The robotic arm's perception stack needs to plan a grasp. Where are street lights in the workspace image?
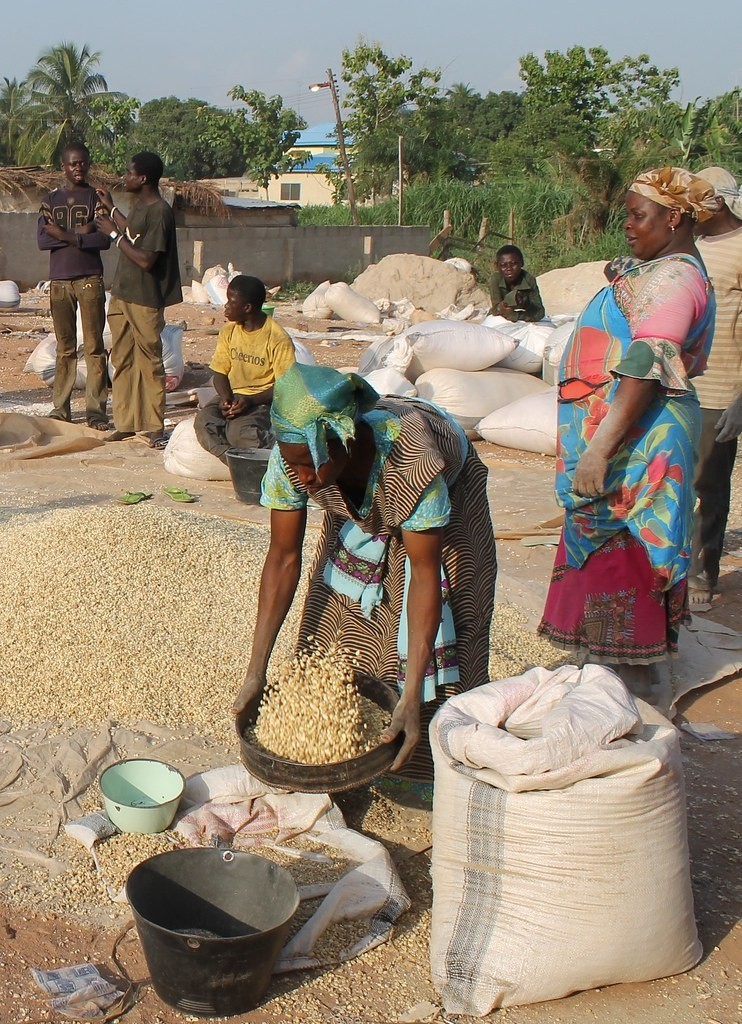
[308,69,360,227]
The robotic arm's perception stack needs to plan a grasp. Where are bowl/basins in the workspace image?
[236,675,405,793]
[98,759,186,835]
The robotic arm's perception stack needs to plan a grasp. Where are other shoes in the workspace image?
[89,420,111,431]
[102,431,136,442]
[150,434,169,450]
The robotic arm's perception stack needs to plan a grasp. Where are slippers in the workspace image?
[159,485,195,502]
[115,491,153,505]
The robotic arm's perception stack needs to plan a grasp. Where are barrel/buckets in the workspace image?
[225,448,273,504]
[262,305,275,318]
[125,849,300,1017]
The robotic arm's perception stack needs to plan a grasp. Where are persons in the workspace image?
[194,275,296,466]
[689,165,742,604]
[234,362,498,782]
[96,152,183,449]
[536,167,716,697]
[486,245,545,323]
[37,142,111,431]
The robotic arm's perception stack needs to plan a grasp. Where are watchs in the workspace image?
[108,229,118,242]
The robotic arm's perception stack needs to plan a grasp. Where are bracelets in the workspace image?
[111,207,117,218]
[115,235,123,247]
